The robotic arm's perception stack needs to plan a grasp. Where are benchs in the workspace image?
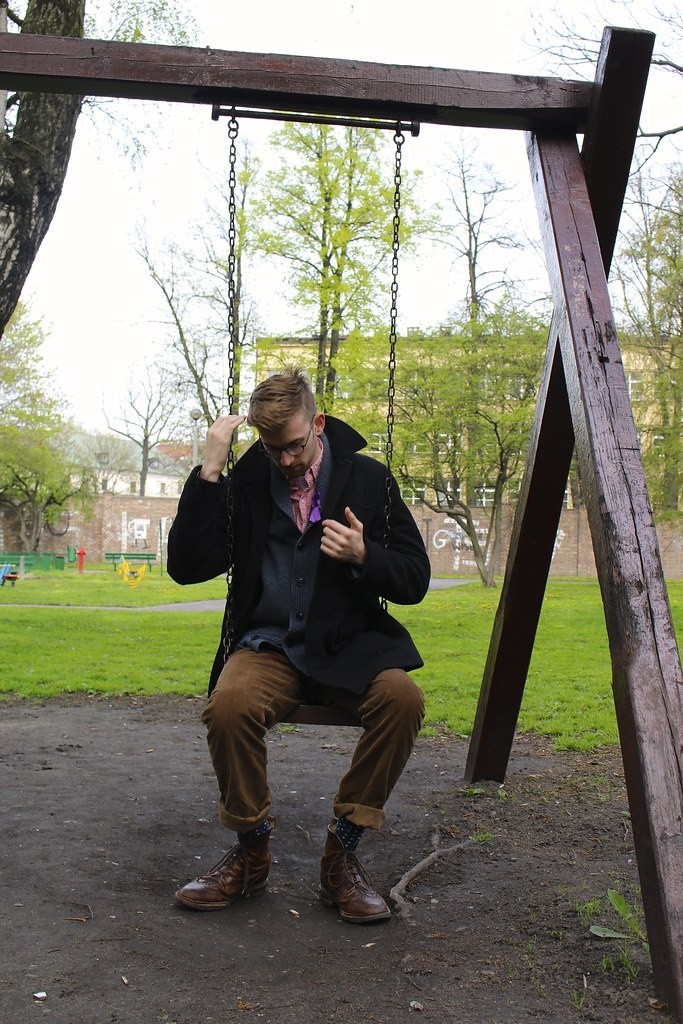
[104,552,157,573]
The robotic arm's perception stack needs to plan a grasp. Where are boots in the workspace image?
[319,815,391,922]
[172,814,276,910]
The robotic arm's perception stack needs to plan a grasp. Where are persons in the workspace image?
[164,366,432,924]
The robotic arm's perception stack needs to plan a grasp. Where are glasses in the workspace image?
[258,413,316,457]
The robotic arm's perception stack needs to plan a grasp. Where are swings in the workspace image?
[222,117,406,727]
